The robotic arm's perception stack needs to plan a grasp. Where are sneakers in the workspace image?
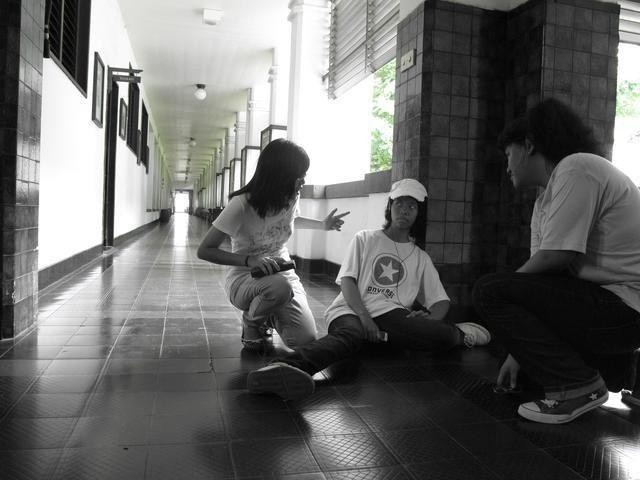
[455,322,492,349]
[517,369,609,424]
[247,362,316,400]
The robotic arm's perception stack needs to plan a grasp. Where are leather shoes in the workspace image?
[260,324,272,336]
[241,316,265,352]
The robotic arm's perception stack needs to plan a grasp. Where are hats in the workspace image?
[390,179,428,202]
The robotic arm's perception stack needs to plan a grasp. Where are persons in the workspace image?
[197,137,351,350]
[470,97,640,426]
[247,177,492,400]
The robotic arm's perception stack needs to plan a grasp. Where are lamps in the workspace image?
[194,84,207,100]
[189,137,196,147]
[187,159,192,165]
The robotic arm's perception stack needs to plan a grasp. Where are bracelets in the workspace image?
[245,255,250,266]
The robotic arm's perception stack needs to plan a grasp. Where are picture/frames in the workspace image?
[146,146,149,174]
[92,51,105,128]
[137,129,142,165]
[119,98,128,140]
